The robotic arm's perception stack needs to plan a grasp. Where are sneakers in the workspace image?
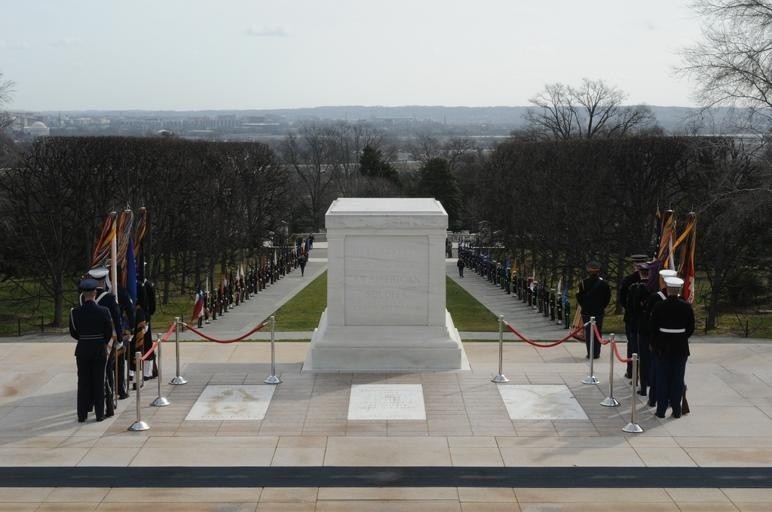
[77,368,158,423]
[624,372,681,418]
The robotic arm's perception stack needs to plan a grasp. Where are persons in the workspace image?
[67,260,160,421]
[194,248,309,328]
[457,244,571,329]
[309,232,314,250]
[619,253,697,419]
[575,259,612,358]
[447,241,452,258]
[446,237,449,246]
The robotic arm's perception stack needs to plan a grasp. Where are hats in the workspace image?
[79,278,99,292]
[586,261,602,273]
[662,276,684,289]
[658,269,678,278]
[88,268,110,280]
[637,263,651,271]
[630,254,649,262]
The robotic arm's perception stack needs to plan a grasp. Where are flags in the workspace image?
[89,206,148,301]
[654,207,696,304]
